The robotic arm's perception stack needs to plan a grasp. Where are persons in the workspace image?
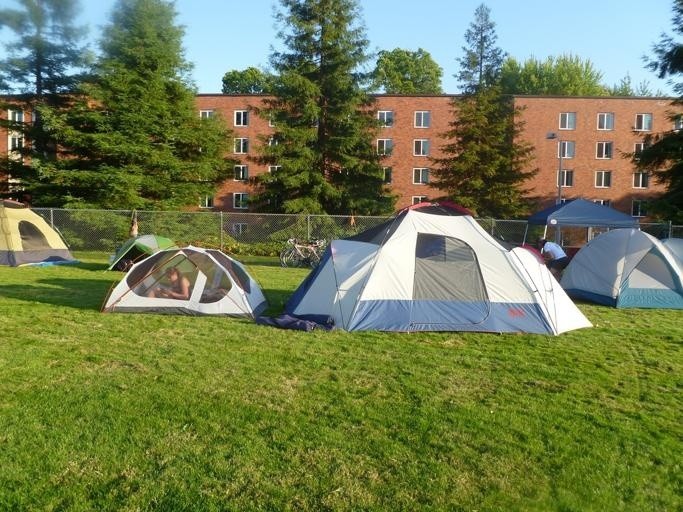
[154,265,226,299]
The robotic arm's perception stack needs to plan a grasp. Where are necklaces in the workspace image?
[537,237,570,275]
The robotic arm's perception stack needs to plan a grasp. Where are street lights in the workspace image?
[544,131,562,244]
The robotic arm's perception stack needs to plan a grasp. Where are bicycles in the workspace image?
[279,237,326,269]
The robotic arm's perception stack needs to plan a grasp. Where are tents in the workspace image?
[282,202,593,337]
[559,227,683,308]
[0,198,74,266]
[100,244,270,320]
[108,233,178,271]
